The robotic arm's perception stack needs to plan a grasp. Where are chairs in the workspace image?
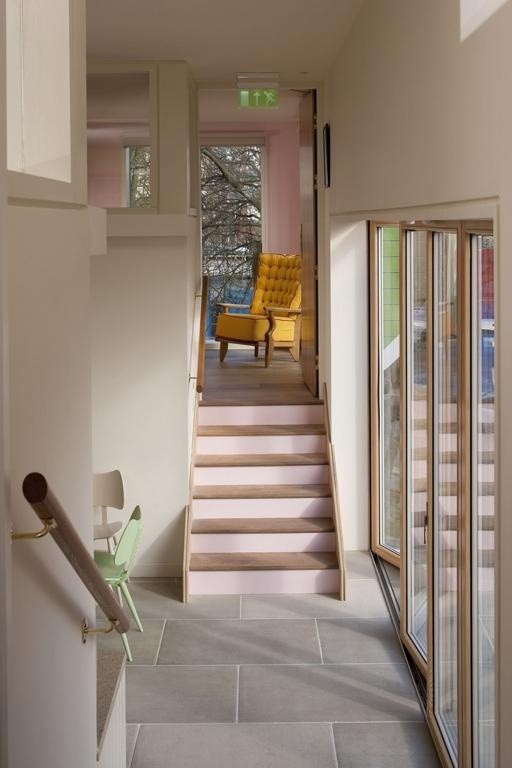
[214,253,302,367]
[94,470,144,663]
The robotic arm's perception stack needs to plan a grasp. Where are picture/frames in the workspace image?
[323,124,330,187]
